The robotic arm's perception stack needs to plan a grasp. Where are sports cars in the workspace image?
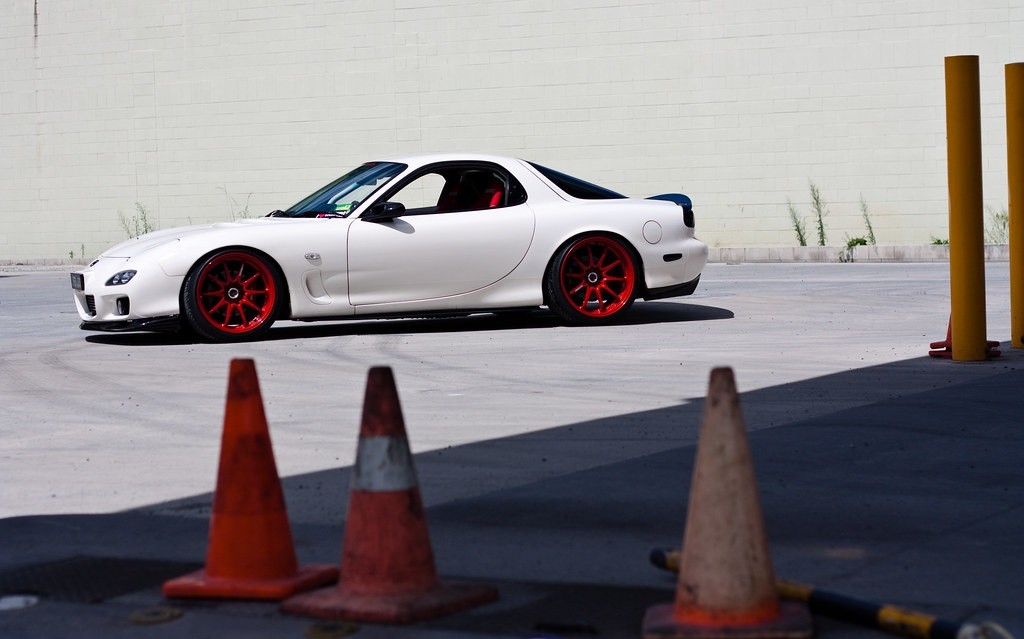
[70,154,709,342]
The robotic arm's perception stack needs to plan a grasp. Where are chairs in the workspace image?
[477,183,501,207]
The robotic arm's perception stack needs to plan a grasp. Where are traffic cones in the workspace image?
[278,365,498,626]
[641,365,814,639]
[161,358,339,601]
